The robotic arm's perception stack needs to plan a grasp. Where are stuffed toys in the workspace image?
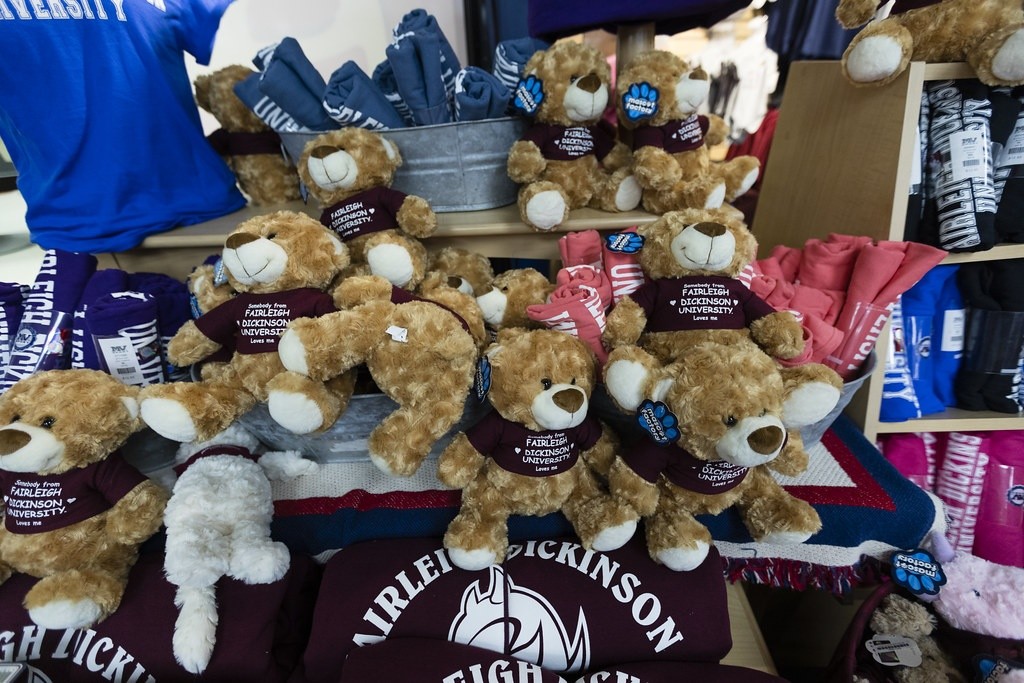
[2,41,843,675]
[835,0,1024,82]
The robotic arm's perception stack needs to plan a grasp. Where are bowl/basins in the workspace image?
[234,391,476,463]
[802,346,880,455]
[272,115,533,213]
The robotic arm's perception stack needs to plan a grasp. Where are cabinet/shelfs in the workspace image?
[90,56,1024,683]
